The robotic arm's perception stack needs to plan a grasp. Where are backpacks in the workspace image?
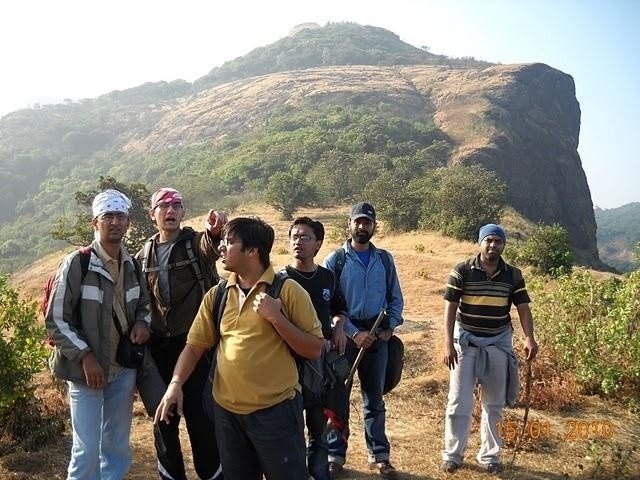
[212,274,325,409]
[41,247,91,347]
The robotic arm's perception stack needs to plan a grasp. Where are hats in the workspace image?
[478,223,506,245]
[152,187,183,209]
[92,189,132,219]
[350,202,376,223]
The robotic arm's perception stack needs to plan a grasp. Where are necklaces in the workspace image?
[289,264,319,280]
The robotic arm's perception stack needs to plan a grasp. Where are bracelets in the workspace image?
[351,330,360,338]
[170,380,182,386]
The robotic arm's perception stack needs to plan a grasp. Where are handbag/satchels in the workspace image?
[325,347,353,393]
[110,304,144,369]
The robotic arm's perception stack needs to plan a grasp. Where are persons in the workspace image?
[153,217,324,480]
[441,224,538,475]
[136,188,228,480]
[322,203,404,479]
[45,191,151,480]
[276,216,349,480]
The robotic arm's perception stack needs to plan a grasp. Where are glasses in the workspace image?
[95,212,127,222]
[153,202,182,209]
[219,238,243,246]
[291,234,317,241]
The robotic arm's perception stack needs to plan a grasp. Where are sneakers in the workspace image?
[369,461,396,477]
[441,461,457,472]
[485,462,501,472]
[329,462,343,478]
